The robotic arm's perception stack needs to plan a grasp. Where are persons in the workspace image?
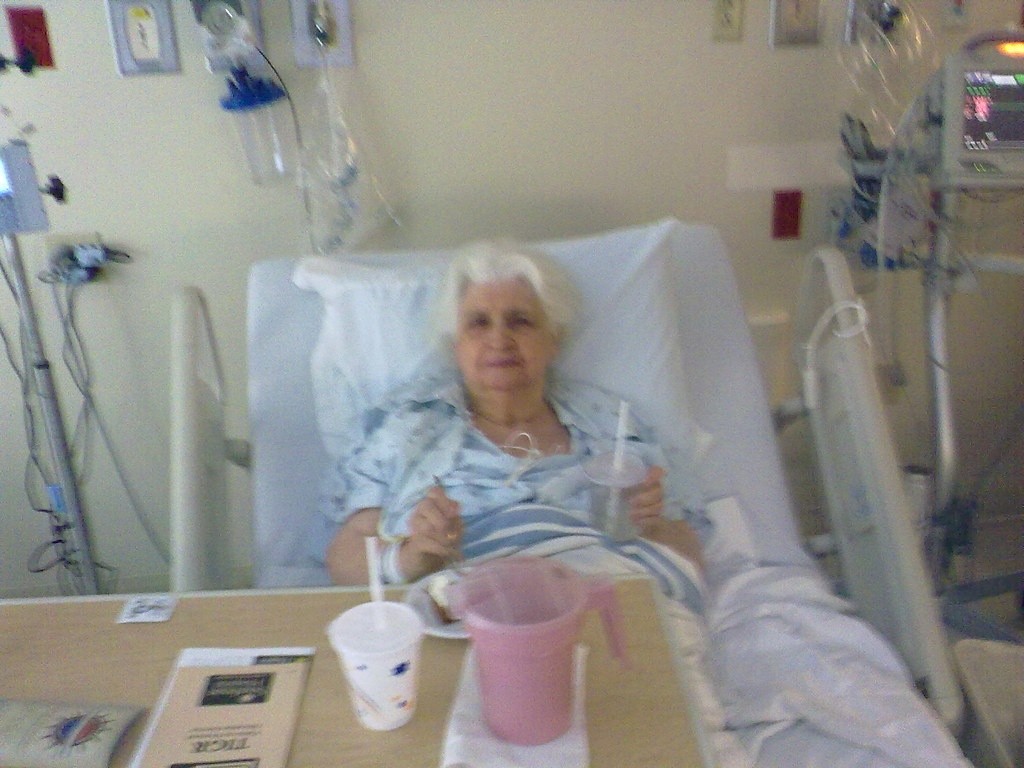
[318,236,706,584]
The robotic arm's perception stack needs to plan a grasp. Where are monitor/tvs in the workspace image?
[943,51,1024,193]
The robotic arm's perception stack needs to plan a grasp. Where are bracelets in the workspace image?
[380,532,409,586]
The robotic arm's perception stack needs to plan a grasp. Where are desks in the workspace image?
[0,574,703,767]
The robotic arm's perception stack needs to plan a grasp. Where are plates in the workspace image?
[407,566,480,640]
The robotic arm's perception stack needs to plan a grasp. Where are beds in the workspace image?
[166,216,977,768]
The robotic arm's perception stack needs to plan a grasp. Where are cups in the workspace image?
[584,450,648,541]
[456,557,634,746]
[326,599,423,733]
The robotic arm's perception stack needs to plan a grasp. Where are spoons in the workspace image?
[428,472,467,575]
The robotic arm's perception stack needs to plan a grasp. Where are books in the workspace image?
[129,646,317,768]
